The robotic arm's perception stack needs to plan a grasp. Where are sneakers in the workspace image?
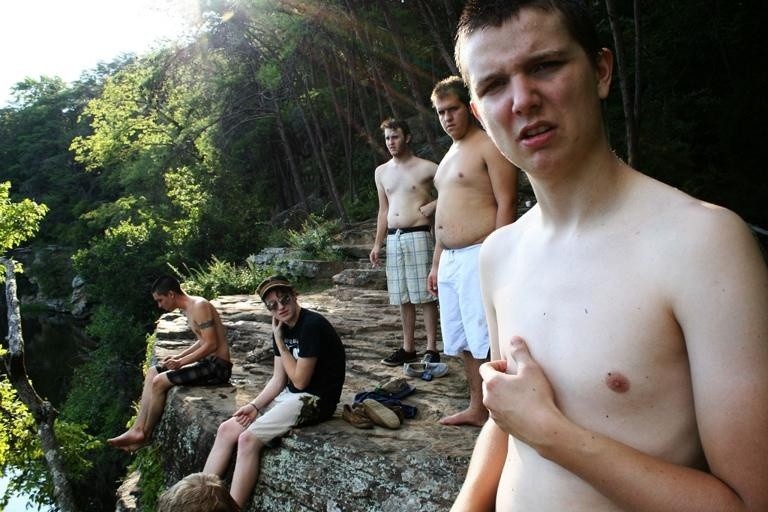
[342,404,373,429]
[406,363,448,378]
[363,399,400,430]
[381,348,417,367]
[423,351,440,364]
[387,406,403,425]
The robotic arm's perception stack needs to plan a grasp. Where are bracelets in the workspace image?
[248,403,259,412]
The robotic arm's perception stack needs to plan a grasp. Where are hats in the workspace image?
[376,377,416,401]
[256,275,292,300]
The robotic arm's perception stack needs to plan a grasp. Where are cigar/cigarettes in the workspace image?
[371,261,378,270]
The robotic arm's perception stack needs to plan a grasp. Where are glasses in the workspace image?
[265,296,291,312]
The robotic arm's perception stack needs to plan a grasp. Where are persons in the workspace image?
[107,275,233,451]
[369,119,440,366]
[154,472,242,512]
[447,1,768,512]
[427,76,518,427]
[203,276,345,510]
